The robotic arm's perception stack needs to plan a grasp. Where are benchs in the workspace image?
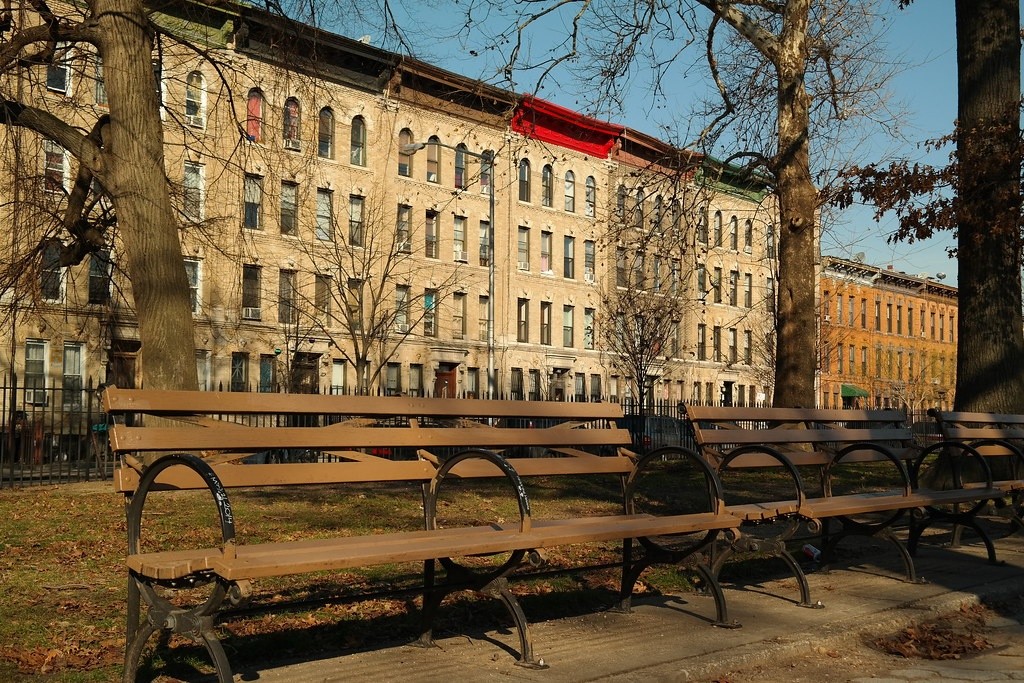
[927,408,1024,567]
[103,385,742,683]
[677,403,1008,608]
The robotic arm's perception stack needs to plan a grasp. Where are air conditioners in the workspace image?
[396,243,413,254]
[395,324,409,333]
[243,307,262,320]
[282,139,301,150]
[454,252,467,262]
[518,261,530,272]
[26,390,49,405]
[186,114,205,129]
[585,273,597,283]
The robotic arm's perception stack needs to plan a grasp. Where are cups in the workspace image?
[802,544,821,562]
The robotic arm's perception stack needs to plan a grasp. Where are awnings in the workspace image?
[841,384,869,398]
